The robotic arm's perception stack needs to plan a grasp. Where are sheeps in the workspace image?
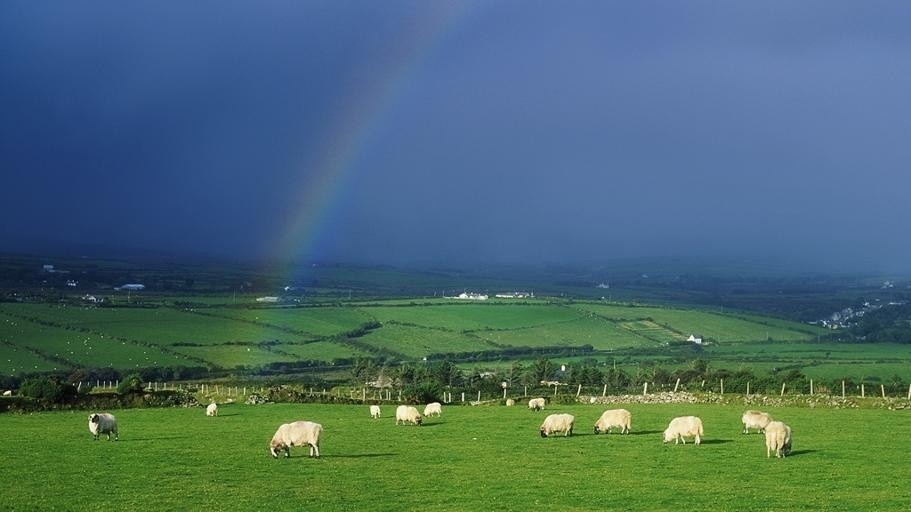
[206,402,219,417]
[269,420,324,459]
[741,410,774,434]
[421,402,442,418]
[87,413,118,441]
[765,420,792,458]
[395,405,423,427]
[370,405,381,419]
[528,397,546,411]
[662,415,704,446]
[506,398,515,407]
[593,408,632,435]
[539,413,575,438]
